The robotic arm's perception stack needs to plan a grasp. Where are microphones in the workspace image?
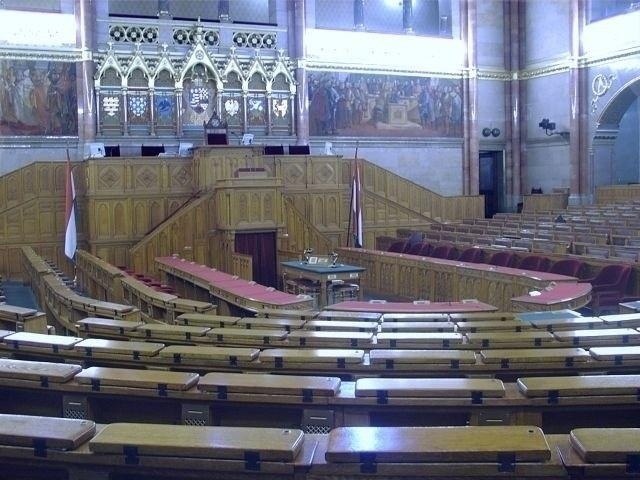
[231,132,240,145]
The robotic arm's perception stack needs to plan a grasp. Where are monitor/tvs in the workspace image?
[83,143,106,159]
[241,134,254,145]
[324,141,333,155]
[178,143,194,157]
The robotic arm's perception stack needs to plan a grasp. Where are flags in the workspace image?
[64,159,77,259]
[352,161,363,247]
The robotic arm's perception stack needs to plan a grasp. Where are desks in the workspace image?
[0,246,640,480]
[375,199,640,246]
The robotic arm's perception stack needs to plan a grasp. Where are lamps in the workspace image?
[538,117,570,138]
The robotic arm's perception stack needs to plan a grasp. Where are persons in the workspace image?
[2,59,77,135]
[306,71,463,139]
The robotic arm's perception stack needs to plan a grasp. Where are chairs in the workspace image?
[140,142,165,157]
[264,144,310,156]
[103,145,121,157]
[206,132,229,147]
